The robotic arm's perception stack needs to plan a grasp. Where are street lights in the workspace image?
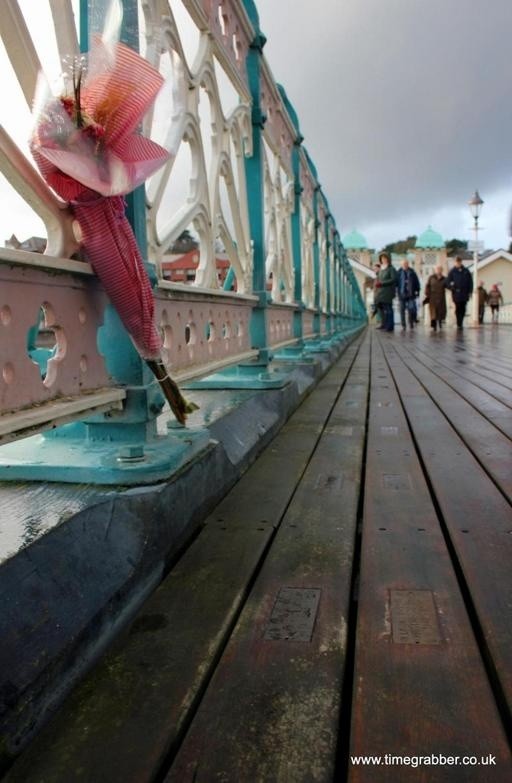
[466,190,486,328]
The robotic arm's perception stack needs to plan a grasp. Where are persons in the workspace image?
[371,250,397,332]
[486,284,504,323]
[423,265,461,333]
[477,280,488,324]
[446,253,473,330]
[394,257,421,332]
[372,263,384,329]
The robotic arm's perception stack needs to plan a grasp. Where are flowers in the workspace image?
[31,55,200,425]
